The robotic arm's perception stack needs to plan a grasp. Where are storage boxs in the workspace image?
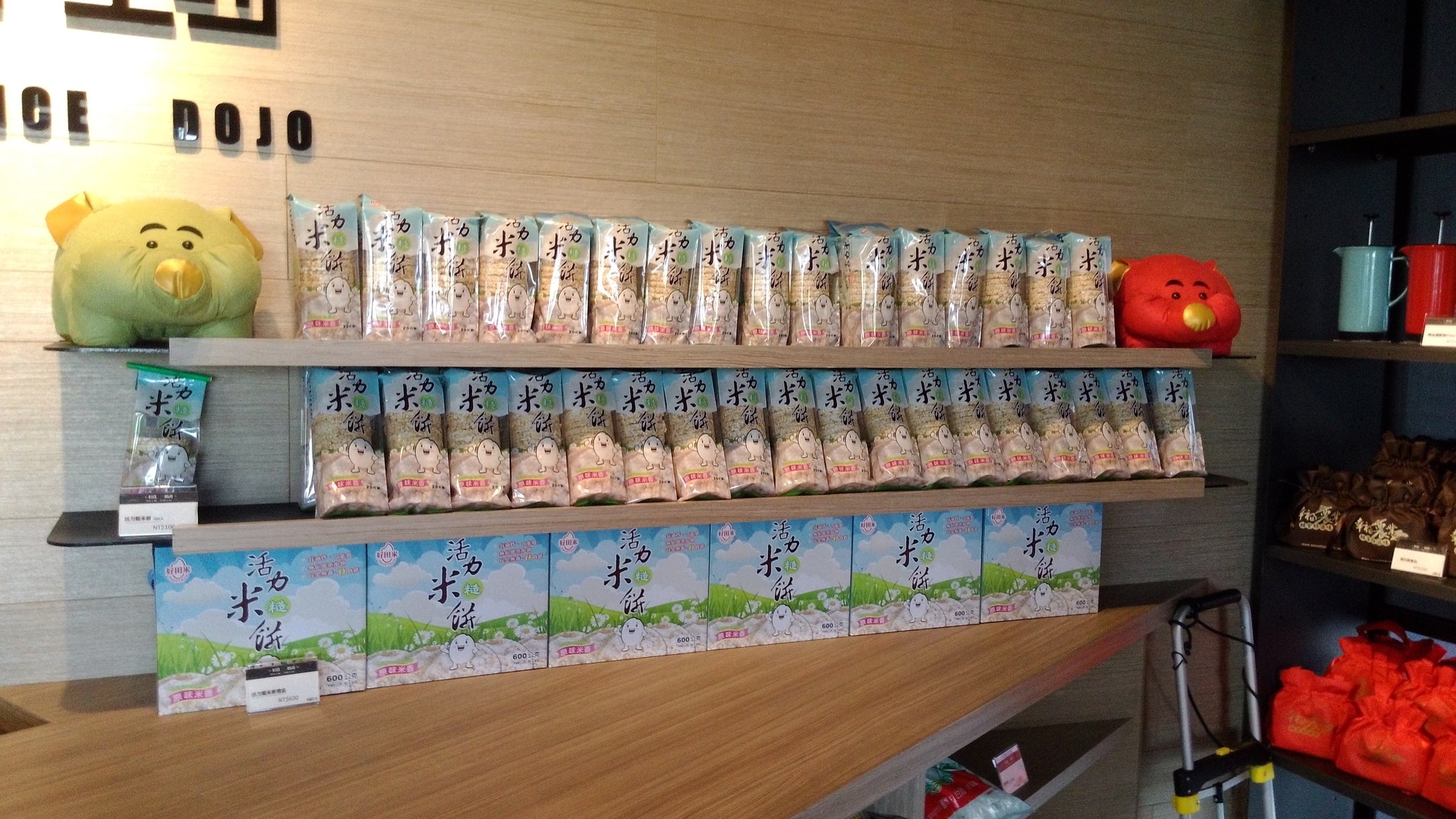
[151,539,368,715]
[366,535,551,693]
[848,508,983,638]
[981,503,1103,624]
[708,517,854,652]
[549,524,709,670]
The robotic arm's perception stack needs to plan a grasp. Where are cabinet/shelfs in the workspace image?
[1,577,1210,819]
[45,331,1259,555]
[1258,109,1456,819]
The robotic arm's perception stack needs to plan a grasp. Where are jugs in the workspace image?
[1400,245,1455,335]
[1333,245,1408,334]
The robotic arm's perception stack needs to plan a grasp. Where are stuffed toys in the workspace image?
[49,189,265,346]
[1110,254,1241,356]
